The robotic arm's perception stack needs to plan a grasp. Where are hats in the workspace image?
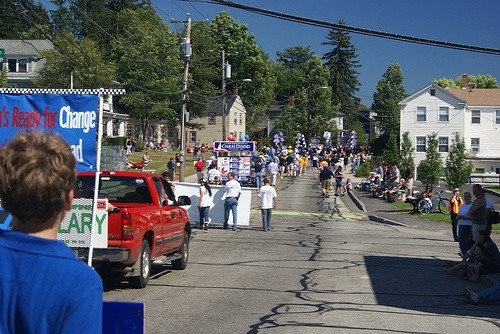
[453,188,460,192]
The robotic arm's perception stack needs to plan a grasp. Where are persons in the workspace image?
[187,140,414,201]
[223,172,241,231]
[259,178,278,232]
[141,152,152,164]
[464,281,500,305]
[162,171,175,194]
[0,128,104,334]
[417,188,433,212]
[444,184,500,281]
[175,153,180,172]
[197,178,212,230]
[148,139,180,151]
[126,136,137,154]
[125,157,136,164]
[167,158,176,181]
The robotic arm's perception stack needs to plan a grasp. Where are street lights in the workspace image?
[221,78,253,141]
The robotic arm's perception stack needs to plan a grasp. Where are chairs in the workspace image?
[124,192,142,199]
[136,186,150,199]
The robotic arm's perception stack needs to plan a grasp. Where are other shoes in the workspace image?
[469,274,479,282]
[223,226,228,229]
[463,275,471,279]
[199,226,203,229]
[232,228,237,231]
[264,228,272,232]
[446,268,456,273]
[204,225,208,230]
[341,194,345,196]
[334,193,339,196]
[458,273,466,278]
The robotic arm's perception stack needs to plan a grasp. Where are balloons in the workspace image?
[262,162,266,166]
[245,135,249,139]
[240,137,243,141]
[227,136,236,141]
[267,157,271,161]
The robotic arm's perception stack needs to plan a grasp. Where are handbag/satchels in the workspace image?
[489,211,500,224]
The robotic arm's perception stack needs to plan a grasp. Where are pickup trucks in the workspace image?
[54,170,193,290]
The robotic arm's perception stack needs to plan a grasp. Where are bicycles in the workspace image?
[417,190,451,216]
